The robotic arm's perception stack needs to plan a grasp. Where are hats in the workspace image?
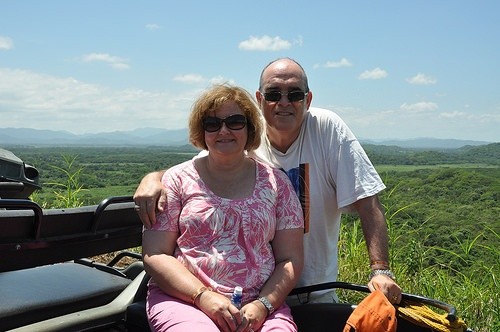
[342,289,397,332]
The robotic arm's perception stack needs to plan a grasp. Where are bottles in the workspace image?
[227,285,244,329]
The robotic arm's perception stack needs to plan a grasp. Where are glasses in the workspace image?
[260,90,308,102]
[202,114,247,133]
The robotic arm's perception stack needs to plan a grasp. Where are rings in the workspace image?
[135,204,140,212]
[250,329,254,332]
[392,295,398,300]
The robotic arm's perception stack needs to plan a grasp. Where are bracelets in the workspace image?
[191,287,207,305]
[370,260,389,266]
[256,296,275,316]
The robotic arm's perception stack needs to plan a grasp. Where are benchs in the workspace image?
[0,190,139,330]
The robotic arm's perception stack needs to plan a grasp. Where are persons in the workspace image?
[141,85,305,332]
[132,57,402,306]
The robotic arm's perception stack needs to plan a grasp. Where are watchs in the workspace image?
[369,270,394,279]
